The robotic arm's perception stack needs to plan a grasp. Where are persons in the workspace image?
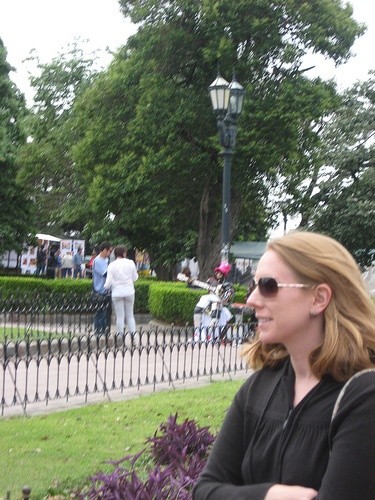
[206,265,235,307]
[91,241,138,336]
[33,242,100,279]
[192,231,375,500]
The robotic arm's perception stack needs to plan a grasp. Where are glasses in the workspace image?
[252,277,313,297]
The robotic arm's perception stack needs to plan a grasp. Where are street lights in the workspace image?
[208,62,246,266]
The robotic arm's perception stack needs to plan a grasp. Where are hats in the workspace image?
[212,261,232,278]
